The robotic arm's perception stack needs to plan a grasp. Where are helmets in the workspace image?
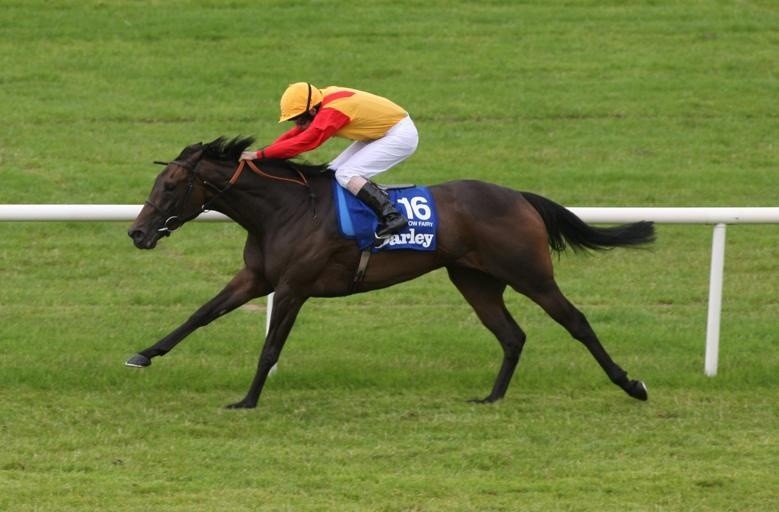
[278,82,323,123]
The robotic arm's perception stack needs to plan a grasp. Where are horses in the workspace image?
[124,134,657,409]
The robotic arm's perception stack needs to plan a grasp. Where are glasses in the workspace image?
[294,83,314,126]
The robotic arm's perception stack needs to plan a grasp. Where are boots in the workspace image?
[356,181,408,237]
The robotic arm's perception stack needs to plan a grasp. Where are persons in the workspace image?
[237,81,419,236]
